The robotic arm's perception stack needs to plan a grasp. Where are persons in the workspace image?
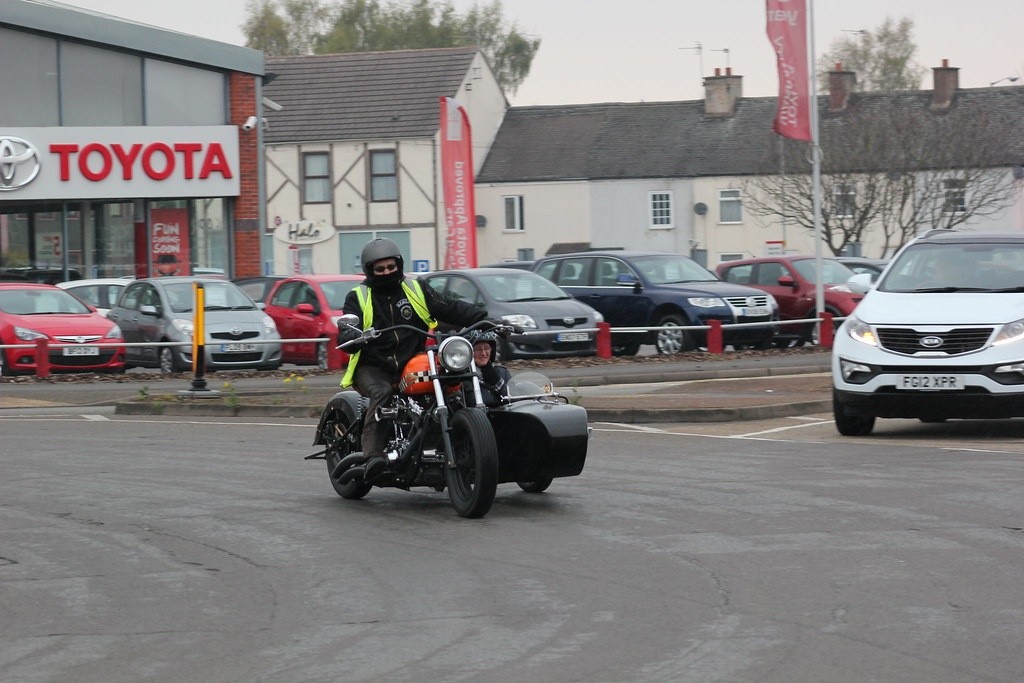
[457,329,513,407]
[335,238,526,480]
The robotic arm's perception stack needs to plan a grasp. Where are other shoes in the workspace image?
[366,457,389,486]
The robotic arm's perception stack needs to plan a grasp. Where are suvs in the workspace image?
[830,228,1024,437]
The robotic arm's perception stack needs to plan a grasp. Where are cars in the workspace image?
[228,277,334,306]
[37,278,156,318]
[822,258,890,282]
[108,275,284,374]
[532,251,779,354]
[716,256,868,347]
[420,268,604,361]
[0,281,127,374]
[265,274,368,372]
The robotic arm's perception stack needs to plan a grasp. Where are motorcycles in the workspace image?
[303,314,592,521]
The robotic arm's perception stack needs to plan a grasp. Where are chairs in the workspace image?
[178,292,191,310]
[560,263,664,283]
[457,281,509,303]
[732,265,785,282]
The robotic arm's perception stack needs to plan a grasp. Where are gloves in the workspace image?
[339,329,368,351]
[481,364,505,392]
[445,371,466,387]
[484,317,524,339]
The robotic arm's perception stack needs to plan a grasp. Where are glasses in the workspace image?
[373,263,398,272]
[474,346,492,354]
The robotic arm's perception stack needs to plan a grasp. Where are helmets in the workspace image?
[361,237,404,281]
[463,330,498,364]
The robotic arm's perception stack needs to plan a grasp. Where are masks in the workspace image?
[371,272,401,289]
